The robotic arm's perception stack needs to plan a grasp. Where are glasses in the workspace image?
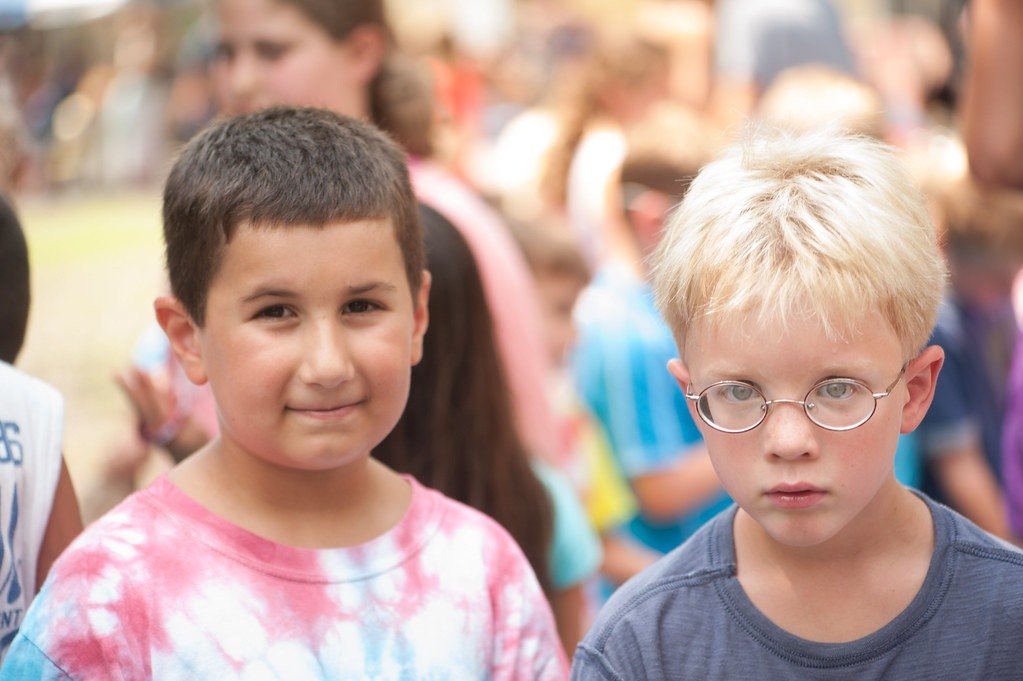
[685,364,908,435]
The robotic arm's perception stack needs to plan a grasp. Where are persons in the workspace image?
[0,0,1023,681]
